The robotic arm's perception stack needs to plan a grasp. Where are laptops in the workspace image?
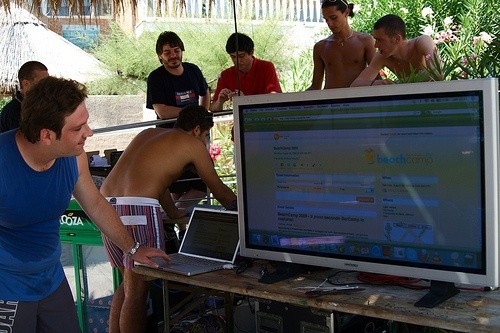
[134,207,241,277]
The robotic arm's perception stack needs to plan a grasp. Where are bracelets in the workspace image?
[127,241,140,256]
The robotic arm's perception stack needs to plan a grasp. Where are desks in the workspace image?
[161,204,228,224]
[58,200,123,333]
[133,263,500,333]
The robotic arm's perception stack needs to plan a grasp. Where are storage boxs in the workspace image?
[254,299,391,333]
[70,295,114,333]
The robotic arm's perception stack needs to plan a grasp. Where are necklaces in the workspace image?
[340,30,354,46]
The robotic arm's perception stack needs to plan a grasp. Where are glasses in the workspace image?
[204,112,213,119]
[320,0,348,9]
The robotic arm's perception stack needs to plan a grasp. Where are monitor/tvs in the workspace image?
[231,77,500,309]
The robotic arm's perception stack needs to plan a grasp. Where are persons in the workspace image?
[350,14,443,87]
[306,0,382,91]
[209,32,282,112]
[0,31,240,333]
